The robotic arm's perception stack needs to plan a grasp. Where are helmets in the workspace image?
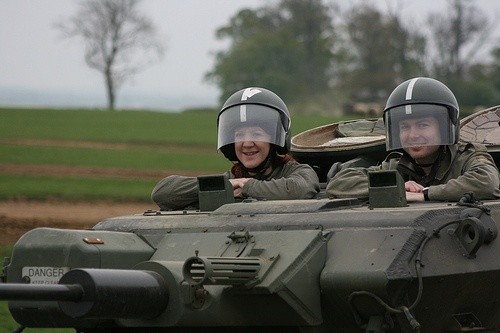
[383,77,460,151]
[217,86,291,161]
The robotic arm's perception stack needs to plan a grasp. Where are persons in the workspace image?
[151,87,319,211]
[325,77,500,202]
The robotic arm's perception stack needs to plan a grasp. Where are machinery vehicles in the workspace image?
[0,103,500,333]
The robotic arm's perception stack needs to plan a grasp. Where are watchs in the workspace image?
[422,187,430,201]
